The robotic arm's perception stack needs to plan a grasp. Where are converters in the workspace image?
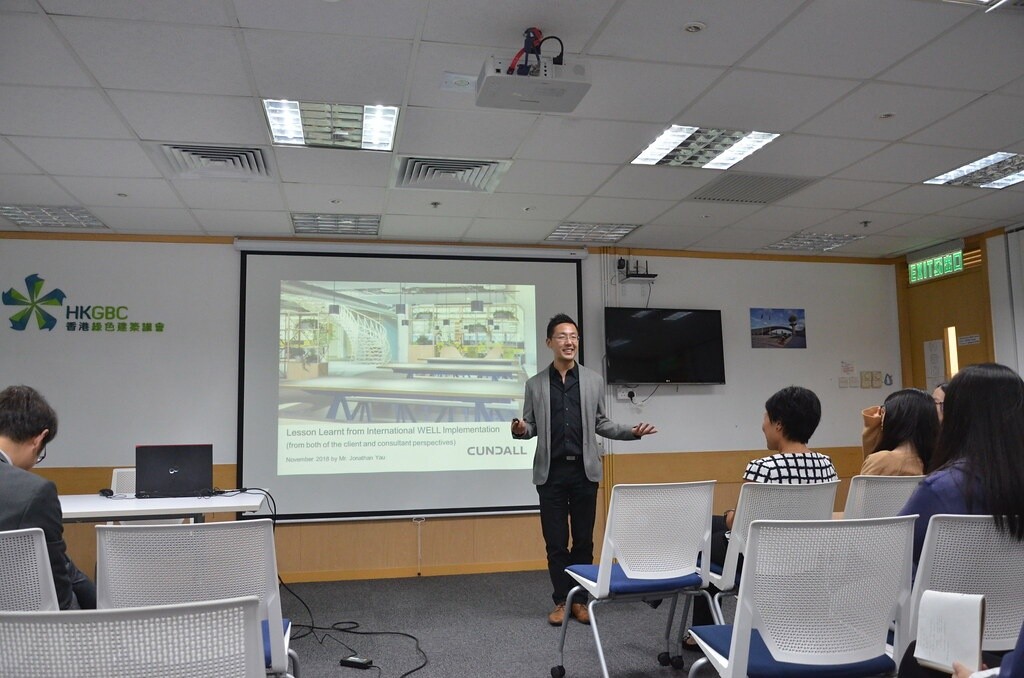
[339,656,372,668]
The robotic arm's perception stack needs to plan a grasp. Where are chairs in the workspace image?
[0,519,301,678]
[105,468,195,524]
[551,475,1023,678]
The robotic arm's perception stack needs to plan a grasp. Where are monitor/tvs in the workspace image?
[604,307,725,385]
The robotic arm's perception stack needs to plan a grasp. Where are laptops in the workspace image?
[135,445,225,499]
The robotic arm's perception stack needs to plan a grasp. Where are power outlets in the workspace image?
[616,388,634,400]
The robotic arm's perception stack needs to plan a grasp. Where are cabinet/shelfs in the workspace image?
[281,310,325,377]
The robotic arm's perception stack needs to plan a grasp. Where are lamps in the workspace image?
[329,280,501,331]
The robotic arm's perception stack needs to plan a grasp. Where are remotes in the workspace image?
[513,418,519,423]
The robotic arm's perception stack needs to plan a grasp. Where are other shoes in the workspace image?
[682,634,701,651]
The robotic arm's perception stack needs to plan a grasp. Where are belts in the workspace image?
[563,455,580,461]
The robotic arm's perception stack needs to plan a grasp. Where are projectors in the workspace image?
[473,54,591,113]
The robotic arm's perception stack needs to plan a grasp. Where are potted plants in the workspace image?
[416,312,516,346]
[287,354,328,383]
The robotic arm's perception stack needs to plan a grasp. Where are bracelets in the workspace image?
[724,509,735,522]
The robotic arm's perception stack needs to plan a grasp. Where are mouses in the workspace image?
[99,489,114,496]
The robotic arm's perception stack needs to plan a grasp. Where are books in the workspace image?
[913,590,987,676]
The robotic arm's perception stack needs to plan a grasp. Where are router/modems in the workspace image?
[625,259,657,278]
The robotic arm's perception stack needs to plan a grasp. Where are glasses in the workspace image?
[36,441,47,463]
[939,402,943,412]
[550,335,579,342]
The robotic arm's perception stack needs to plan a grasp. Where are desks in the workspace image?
[57,488,270,523]
[284,358,526,423]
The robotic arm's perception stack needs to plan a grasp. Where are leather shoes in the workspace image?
[572,603,596,624]
[548,601,566,625]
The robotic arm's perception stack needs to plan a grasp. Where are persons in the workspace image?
[682,387,837,652]
[897,620,1024,678]
[860,383,949,476]
[895,363,1024,570]
[510,314,658,625]
[0,385,97,611]
